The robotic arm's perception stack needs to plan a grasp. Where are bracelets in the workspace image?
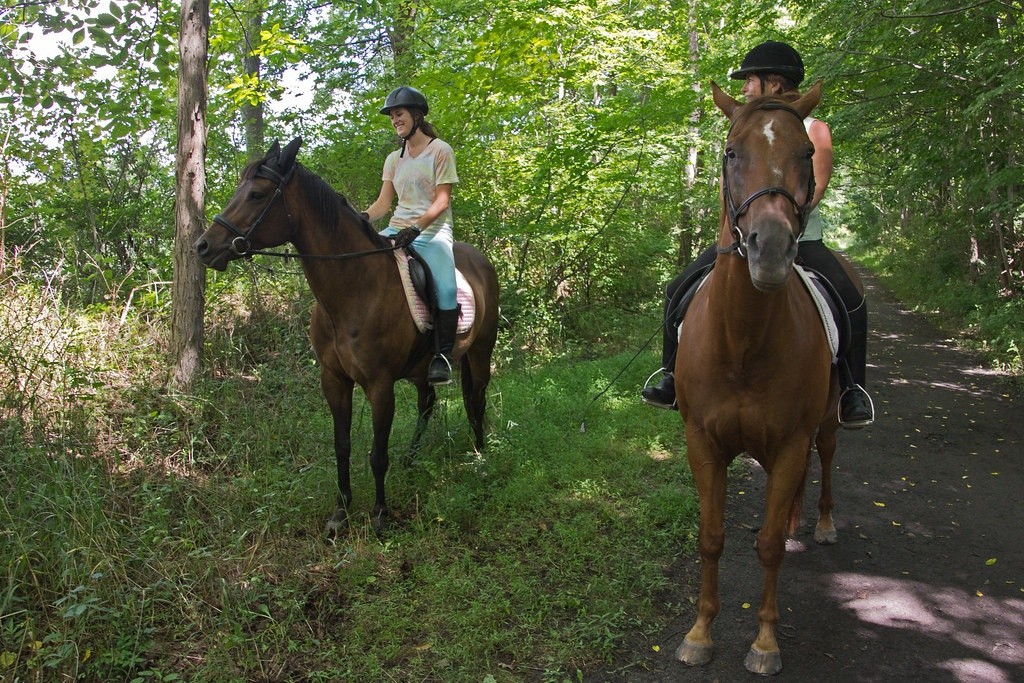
[413,224,423,232]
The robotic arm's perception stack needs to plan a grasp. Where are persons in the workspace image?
[641,39,874,430]
[357,86,461,383]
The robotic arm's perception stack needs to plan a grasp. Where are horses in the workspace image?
[670,75,868,678]
[193,135,501,548]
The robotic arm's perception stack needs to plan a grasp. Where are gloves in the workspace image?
[395,226,421,250]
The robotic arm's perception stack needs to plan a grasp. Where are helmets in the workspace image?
[379,87,429,117]
[729,40,804,82]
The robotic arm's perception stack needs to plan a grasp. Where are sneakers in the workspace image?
[641,374,678,411]
[839,390,871,430]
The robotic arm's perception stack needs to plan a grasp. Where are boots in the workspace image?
[426,303,462,383]
